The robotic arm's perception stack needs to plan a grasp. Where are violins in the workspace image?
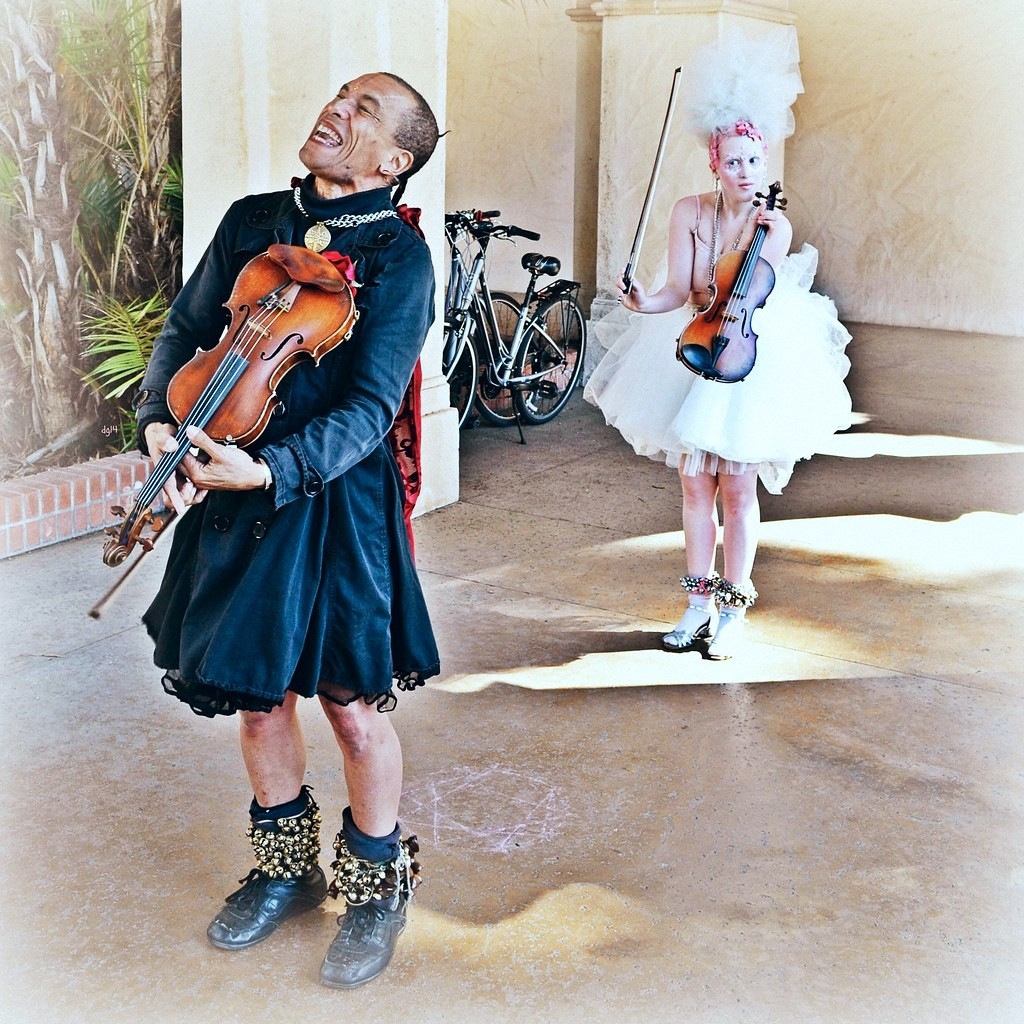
[675,180,788,383]
[103,243,359,571]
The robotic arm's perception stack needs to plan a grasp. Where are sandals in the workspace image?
[710,611,749,661]
[661,604,719,650]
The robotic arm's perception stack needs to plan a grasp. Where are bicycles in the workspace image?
[444,209,587,445]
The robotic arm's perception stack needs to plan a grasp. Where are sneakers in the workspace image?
[319,891,407,990]
[206,867,328,951]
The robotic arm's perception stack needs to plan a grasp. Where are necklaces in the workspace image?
[707,189,759,298]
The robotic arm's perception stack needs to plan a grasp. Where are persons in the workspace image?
[133,73,441,988]
[583,32,853,663]
